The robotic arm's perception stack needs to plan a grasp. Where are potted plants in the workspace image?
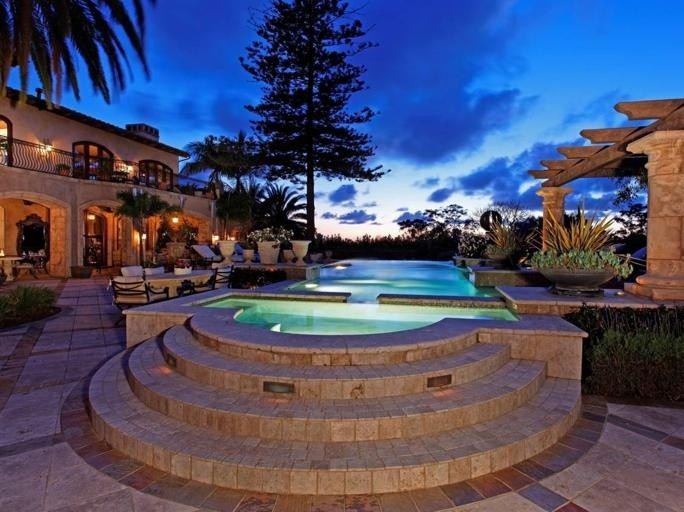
[55,163,71,176]
[483,197,646,291]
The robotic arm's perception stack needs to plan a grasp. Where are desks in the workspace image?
[0,256,24,281]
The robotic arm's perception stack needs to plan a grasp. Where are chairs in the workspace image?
[16,257,39,280]
[110,264,234,326]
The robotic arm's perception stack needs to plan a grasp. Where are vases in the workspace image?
[283,240,312,267]
[256,241,280,264]
[216,240,238,265]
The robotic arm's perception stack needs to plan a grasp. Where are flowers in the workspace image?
[174,260,191,269]
[247,225,295,245]
